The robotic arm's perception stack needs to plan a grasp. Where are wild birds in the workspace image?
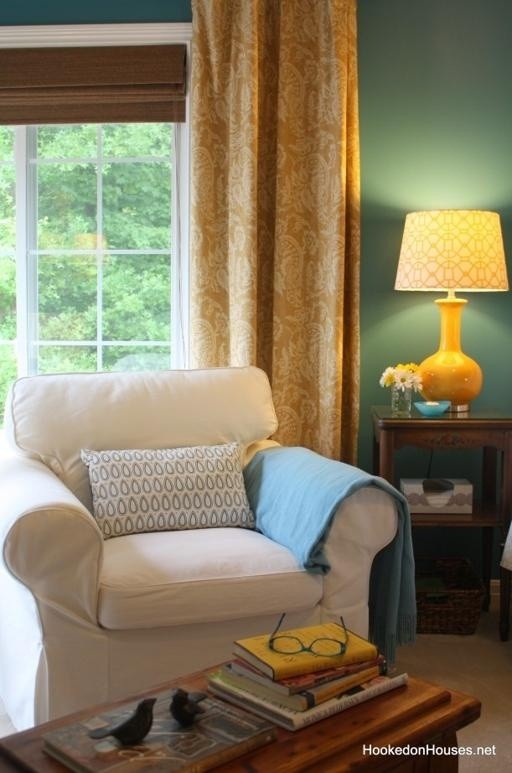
[170,686,209,729]
[89,696,157,744]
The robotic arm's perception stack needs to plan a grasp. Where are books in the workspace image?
[42,687,279,773]
[206,621,410,733]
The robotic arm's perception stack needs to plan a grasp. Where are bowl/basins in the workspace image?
[413,400,452,417]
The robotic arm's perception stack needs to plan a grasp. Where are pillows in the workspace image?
[78,431,254,541]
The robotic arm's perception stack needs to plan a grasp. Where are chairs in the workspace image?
[0,360,410,725]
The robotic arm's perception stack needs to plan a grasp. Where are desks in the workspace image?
[366,403,509,645]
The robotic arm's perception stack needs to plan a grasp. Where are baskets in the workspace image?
[414,557,488,635]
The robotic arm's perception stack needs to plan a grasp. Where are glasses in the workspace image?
[270,614,348,656]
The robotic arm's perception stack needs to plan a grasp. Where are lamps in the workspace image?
[389,205,512,415]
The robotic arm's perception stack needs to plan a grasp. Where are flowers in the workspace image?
[378,361,422,413]
